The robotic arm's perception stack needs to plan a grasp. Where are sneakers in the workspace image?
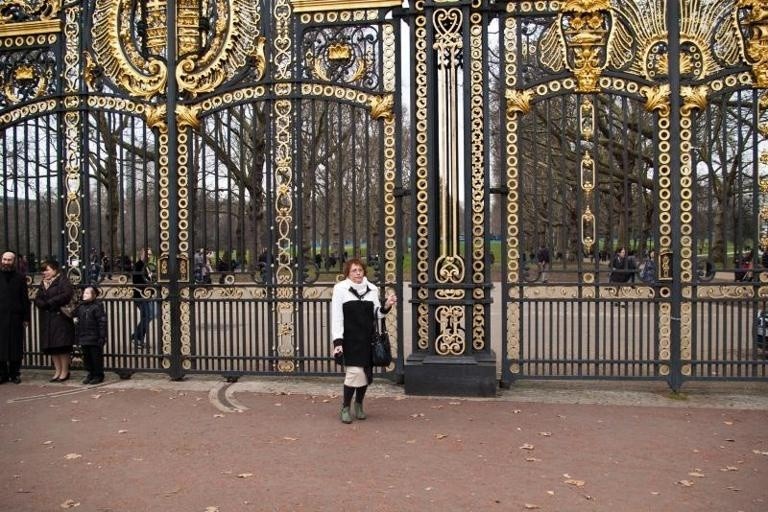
[131,339,147,348]
[82,377,103,384]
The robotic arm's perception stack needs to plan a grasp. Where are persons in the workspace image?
[331,259,398,423]
[0,245,156,385]
[523,240,658,309]
[315,252,348,273]
[734,248,760,282]
[194,247,278,284]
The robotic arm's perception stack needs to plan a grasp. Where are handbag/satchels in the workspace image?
[371,333,392,367]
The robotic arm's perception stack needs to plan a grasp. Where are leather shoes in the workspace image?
[354,399,366,419]
[0,375,21,384]
[48,372,69,382]
[340,403,352,423]
